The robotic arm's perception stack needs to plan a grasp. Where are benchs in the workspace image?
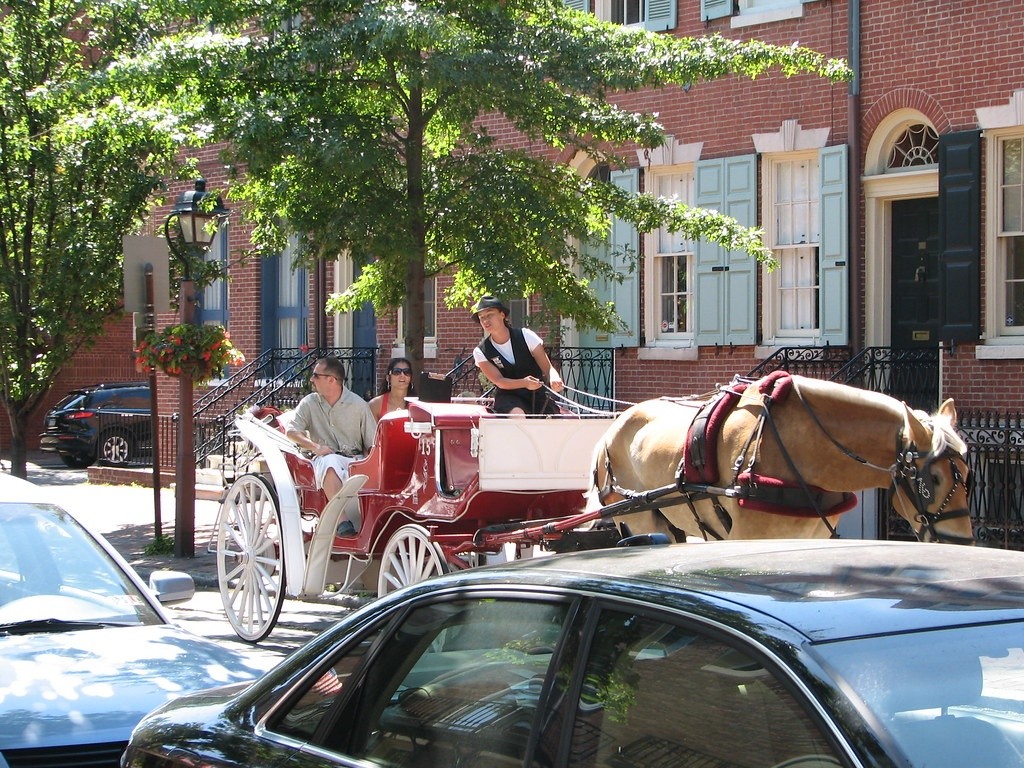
[277,405,479,536]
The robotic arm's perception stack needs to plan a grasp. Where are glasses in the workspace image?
[388,368,412,377]
[312,373,337,380]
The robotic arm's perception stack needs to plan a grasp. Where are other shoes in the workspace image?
[336,520,357,537]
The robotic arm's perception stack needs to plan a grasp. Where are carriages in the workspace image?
[206,369,976,646]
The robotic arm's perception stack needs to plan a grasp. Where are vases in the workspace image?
[164,359,212,379]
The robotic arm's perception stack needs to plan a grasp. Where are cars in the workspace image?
[0,474,275,768]
[118,533,1023,768]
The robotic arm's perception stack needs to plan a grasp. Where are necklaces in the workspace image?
[392,397,404,411]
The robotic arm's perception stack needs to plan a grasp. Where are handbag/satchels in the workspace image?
[418,372,452,403]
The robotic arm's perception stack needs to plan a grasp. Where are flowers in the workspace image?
[296,344,315,396]
[134,324,244,389]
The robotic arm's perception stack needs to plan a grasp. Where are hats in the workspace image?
[470,295,510,324]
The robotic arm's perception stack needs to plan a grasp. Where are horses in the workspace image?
[592,374,977,546]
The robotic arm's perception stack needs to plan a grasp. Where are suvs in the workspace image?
[37,381,151,468]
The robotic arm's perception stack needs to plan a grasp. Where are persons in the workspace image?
[285,357,378,537]
[470,295,564,419]
[368,358,417,425]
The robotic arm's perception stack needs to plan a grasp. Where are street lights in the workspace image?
[164,178,228,558]
